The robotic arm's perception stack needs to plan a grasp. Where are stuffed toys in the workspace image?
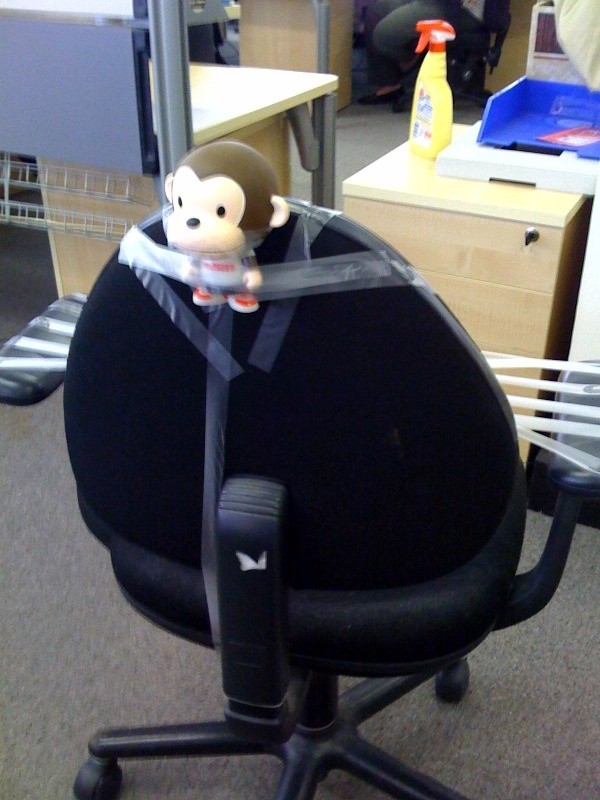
[163,140,290,314]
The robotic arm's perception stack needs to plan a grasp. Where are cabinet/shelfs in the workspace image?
[37,57,340,300]
[341,124,595,473]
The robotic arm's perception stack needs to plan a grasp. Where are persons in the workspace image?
[357,0,500,106]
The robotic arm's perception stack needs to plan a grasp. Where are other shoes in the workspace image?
[392,91,414,111]
[358,85,406,105]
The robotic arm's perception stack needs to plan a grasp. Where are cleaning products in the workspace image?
[405,18,458,165]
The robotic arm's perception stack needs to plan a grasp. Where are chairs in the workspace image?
[0,192,600,800]
